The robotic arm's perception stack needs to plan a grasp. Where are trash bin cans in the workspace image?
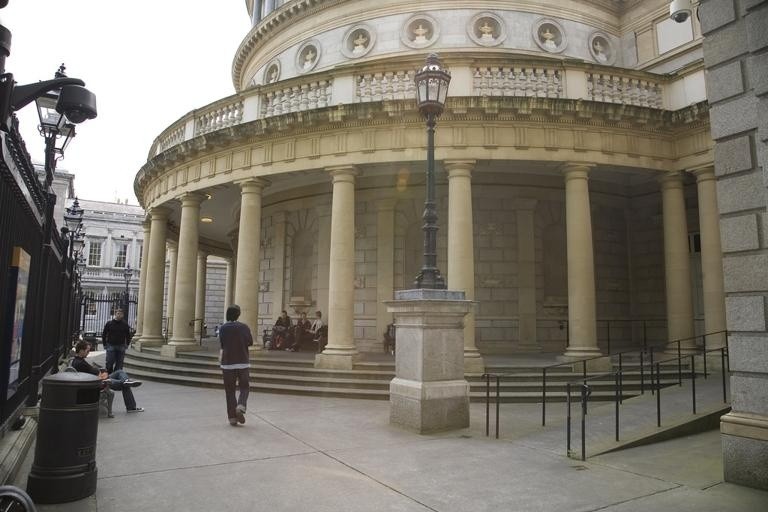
[84,331,96,351]
[26,366,102,504]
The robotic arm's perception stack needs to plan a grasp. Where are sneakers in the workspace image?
[126,405,145,413]
[123,379,142,387]
[229,409,246,426]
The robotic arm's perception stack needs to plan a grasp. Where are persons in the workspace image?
[267,310,291,350]
[71,340,145,413]
[102,305,131,375]
[291,311,323,351]
[286,313,311,351]
[384,318,395,356]
[217,304,253,426]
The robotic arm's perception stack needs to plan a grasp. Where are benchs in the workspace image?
[63,357,115,418]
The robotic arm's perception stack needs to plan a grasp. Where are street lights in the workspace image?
[34,64,77,194]
[63,198,86,275]
[413,53,453,290]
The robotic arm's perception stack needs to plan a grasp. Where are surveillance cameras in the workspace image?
[54,84,98,126]
